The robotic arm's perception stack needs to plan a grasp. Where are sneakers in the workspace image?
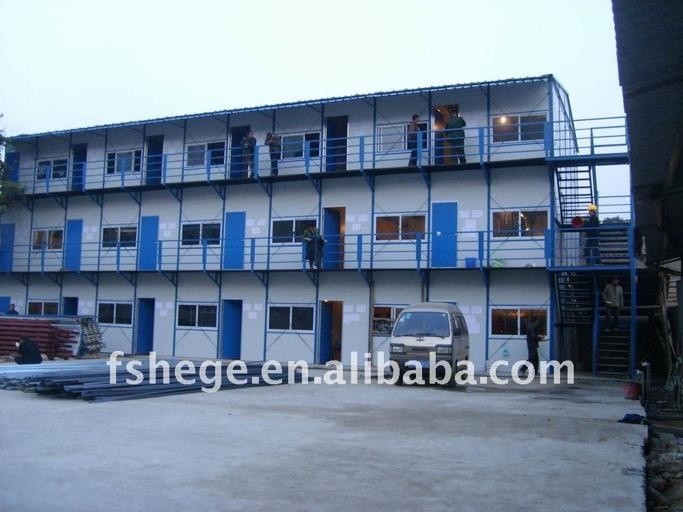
[603,328,619,332]
[518,370,540,377]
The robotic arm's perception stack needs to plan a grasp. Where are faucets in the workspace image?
[640,361,648,366]
[635,369,642,374]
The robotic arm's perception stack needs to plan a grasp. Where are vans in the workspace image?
[389,302,470,388]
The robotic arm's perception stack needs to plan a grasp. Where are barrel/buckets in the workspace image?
[624,381,641,399]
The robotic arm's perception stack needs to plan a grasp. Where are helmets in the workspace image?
[588,204,597,210]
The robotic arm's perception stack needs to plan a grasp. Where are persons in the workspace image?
[518,315,547,378]
[301,220,324,271]
[603,275,625,335]
[264,131,282,176]
[6,303,19,315]
[13,335,44,365]
[408,114,421,166]
[240,130,260,179]
[582,203,603,266]
[444,111,467,165]
